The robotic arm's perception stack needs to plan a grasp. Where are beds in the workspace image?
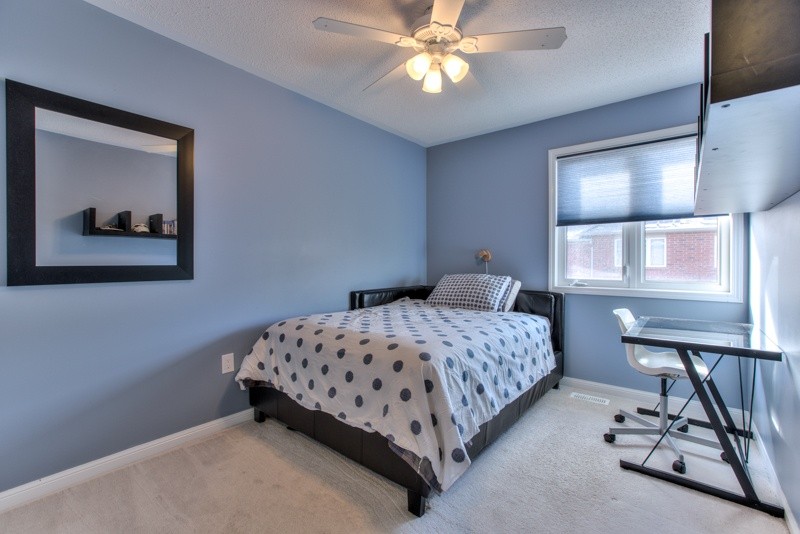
[233,285,564,517]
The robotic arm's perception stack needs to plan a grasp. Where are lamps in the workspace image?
[405,46,469,93]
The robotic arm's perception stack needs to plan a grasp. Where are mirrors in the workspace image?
[4,77,196,286]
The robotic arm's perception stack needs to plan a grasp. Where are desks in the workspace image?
[621,315,786,520]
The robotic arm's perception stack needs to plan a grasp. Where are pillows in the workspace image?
[426,273,522,313]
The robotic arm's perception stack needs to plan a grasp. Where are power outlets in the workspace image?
[221,353,234,374]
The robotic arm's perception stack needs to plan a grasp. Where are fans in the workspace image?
[312,0,568,100]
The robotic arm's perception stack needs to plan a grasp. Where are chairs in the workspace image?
[612,306,736,474]
